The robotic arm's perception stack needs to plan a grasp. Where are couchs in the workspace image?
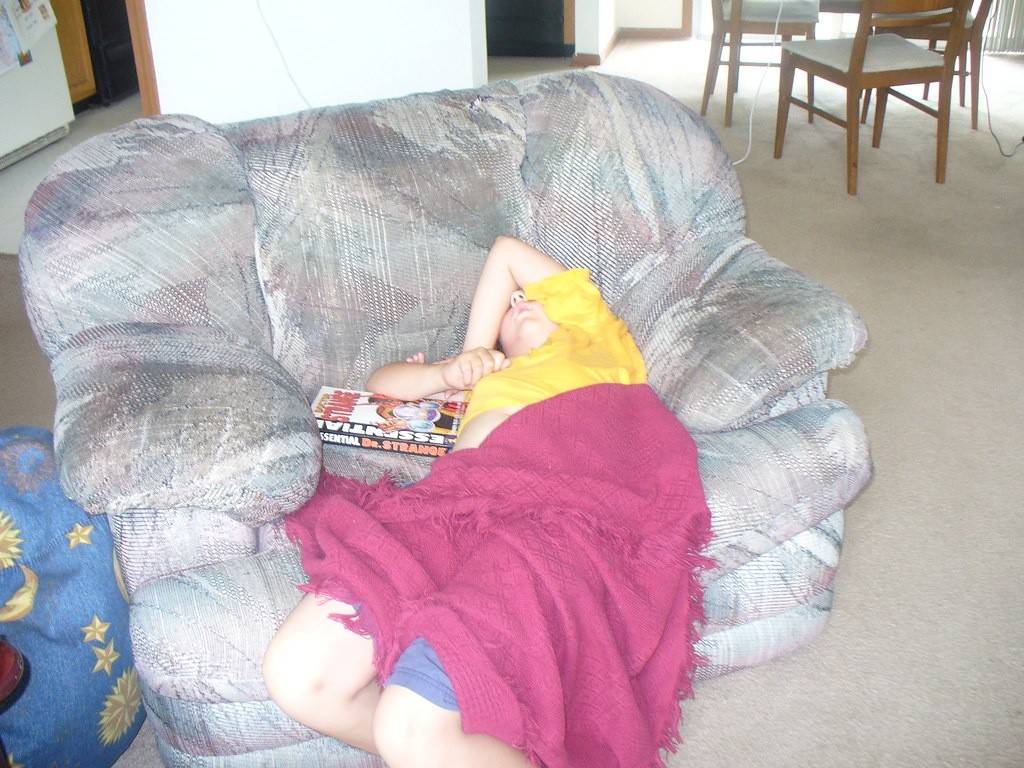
[16,69,872,768]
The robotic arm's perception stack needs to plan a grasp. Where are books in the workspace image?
[311,386,468,457]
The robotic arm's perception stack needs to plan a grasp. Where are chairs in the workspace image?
[861,1,995,131]
[698,0,818,126]
[773,1,973,195]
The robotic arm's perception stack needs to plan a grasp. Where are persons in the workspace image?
[262,235,719,767]
